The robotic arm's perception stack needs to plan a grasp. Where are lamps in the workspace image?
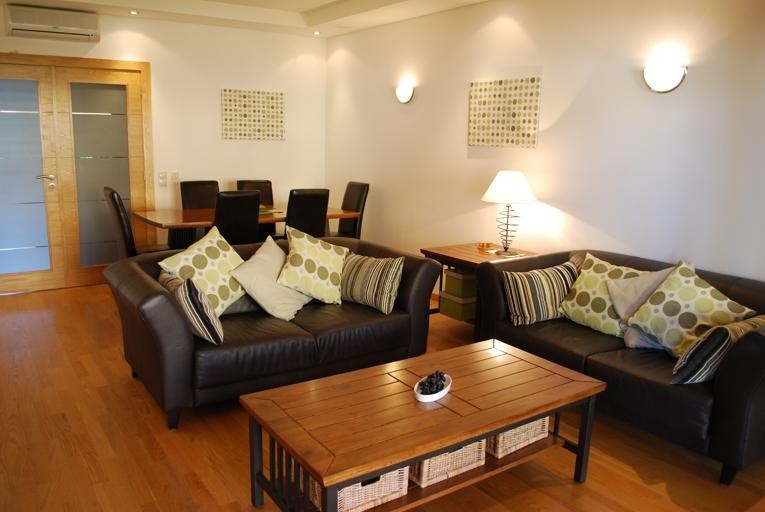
[643,58,686,93]
[480,170,537,257]
[395,82,415,104]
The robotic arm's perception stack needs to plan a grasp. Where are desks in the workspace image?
[132,206,361,248]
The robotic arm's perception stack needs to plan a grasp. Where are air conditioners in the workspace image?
[4,1,101,46]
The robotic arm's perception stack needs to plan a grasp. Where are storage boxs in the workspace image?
[442,269,490,297]
[273,437,410,512]
[438,290,478,321]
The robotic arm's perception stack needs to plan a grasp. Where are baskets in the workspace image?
[290,454,408,511]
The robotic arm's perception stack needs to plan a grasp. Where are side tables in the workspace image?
[421,242,541,341]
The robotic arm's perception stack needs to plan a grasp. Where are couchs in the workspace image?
[479,249,764,487]
[102,235,444,429]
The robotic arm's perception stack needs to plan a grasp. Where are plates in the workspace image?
[413,373,452,403]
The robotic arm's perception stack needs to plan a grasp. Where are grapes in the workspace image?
[419,370,446,395]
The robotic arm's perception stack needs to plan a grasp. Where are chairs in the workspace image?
[284,188,330,239]
[102,186,136,257]
[180,180,219,236]
[331,180,370,239]
[237,179,276,239]
[213,190,260,246]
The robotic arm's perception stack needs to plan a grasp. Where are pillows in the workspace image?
[156,224,247,320]
[556,251,653,338]
[669,314,765,386]
[604,265,677,350]
[500,260,579,328]
[341,252,406,316]
[228,236,317,322]
[628,259,757,357]
[158,269,225,346]
[275,225,351,306]
